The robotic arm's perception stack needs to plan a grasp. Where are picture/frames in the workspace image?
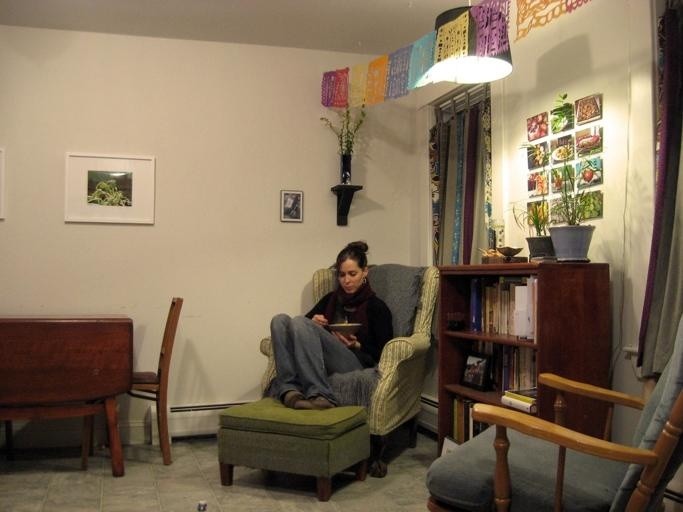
[65,153,156,225]
[281,191,303,222]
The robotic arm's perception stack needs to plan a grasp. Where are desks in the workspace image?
[0,314,135,477]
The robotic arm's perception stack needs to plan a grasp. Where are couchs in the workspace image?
[218,396,371,501]
[261,265,438,477]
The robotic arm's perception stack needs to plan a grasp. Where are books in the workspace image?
[477,340,538,395]
[469,274,539,344]
[452,397,491,444]
[441,436,462,456]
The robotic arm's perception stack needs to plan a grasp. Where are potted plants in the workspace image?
[321,103,366,185]
[521,93,602,262]
[514,197,557,262]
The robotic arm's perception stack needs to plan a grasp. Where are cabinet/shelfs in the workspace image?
[439,262,612,459]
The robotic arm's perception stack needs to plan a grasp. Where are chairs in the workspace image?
[427,312,683,511]
[82,298,184,471]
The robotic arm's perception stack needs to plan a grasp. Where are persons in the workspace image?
[501,388,537,414]
[270,241,394,410]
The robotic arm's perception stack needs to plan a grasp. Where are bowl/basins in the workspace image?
[497,247,522,256]
[328,323,363,336]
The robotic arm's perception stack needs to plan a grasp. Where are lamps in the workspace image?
[430,0,513,84]
